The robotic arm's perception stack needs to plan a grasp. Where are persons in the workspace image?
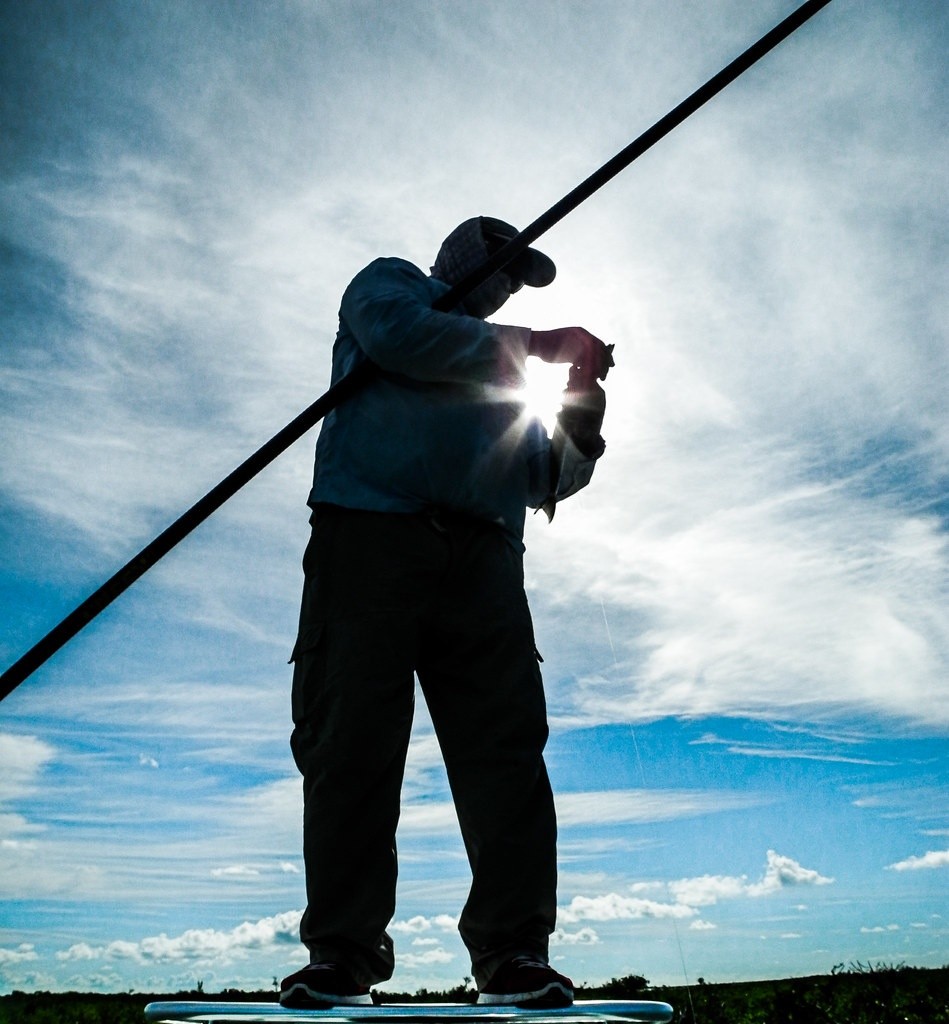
[280,215,615,1009]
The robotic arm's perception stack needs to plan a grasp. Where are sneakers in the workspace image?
[280,963,374,1007]
[477,954,574,1008]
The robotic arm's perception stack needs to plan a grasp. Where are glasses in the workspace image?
[500,264,522,292]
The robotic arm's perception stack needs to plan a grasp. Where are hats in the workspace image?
[444,216,557,287]
